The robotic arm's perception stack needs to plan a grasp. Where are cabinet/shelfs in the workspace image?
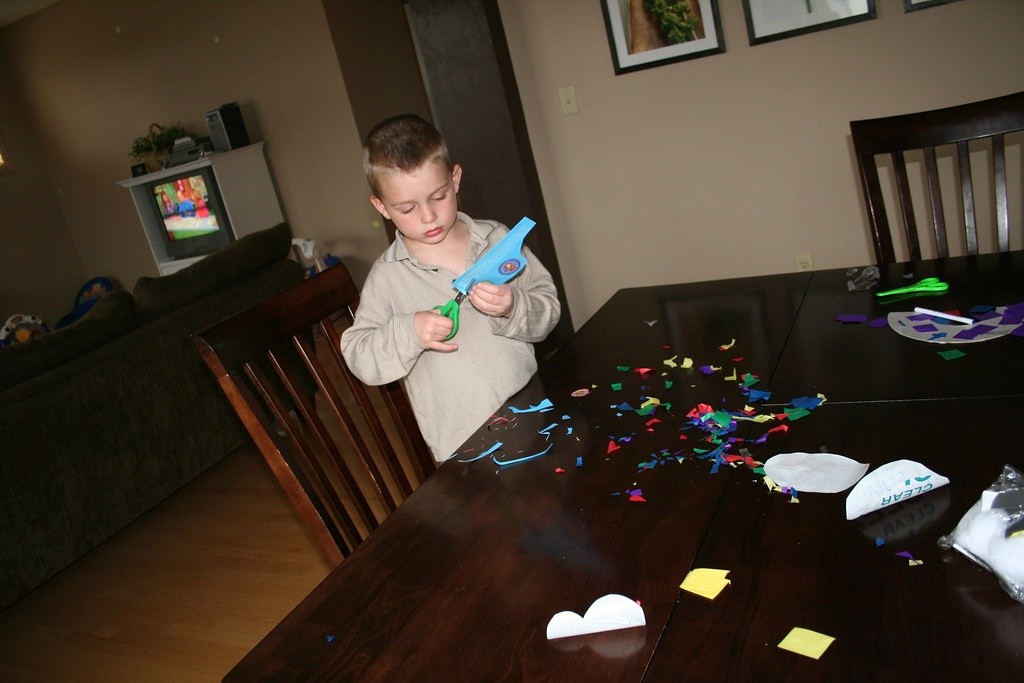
[116,141,286,277]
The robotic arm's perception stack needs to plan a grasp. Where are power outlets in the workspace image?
[793,255,814,272]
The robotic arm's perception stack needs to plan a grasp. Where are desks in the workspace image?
[222,246,1024,683]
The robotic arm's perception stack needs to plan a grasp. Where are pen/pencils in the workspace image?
[914,307,975,326]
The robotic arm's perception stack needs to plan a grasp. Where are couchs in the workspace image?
[0,221,320,610]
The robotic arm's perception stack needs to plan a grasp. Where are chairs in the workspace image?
[849,92,1024,264]
[189,261,436,572]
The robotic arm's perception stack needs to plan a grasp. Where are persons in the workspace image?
[160,189,208,219]
[339,113,561,467]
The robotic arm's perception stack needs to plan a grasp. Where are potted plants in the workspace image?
[130,117,188,172]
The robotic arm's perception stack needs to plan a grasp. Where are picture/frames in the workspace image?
[742,0,877,46]
[600,0,726,75]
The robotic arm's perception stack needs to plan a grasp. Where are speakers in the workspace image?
[205,104,251,151]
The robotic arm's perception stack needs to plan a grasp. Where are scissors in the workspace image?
[431,279,478,342]
[876,277,949,297]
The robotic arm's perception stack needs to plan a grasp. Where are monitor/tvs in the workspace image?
[146,166,236,260]
